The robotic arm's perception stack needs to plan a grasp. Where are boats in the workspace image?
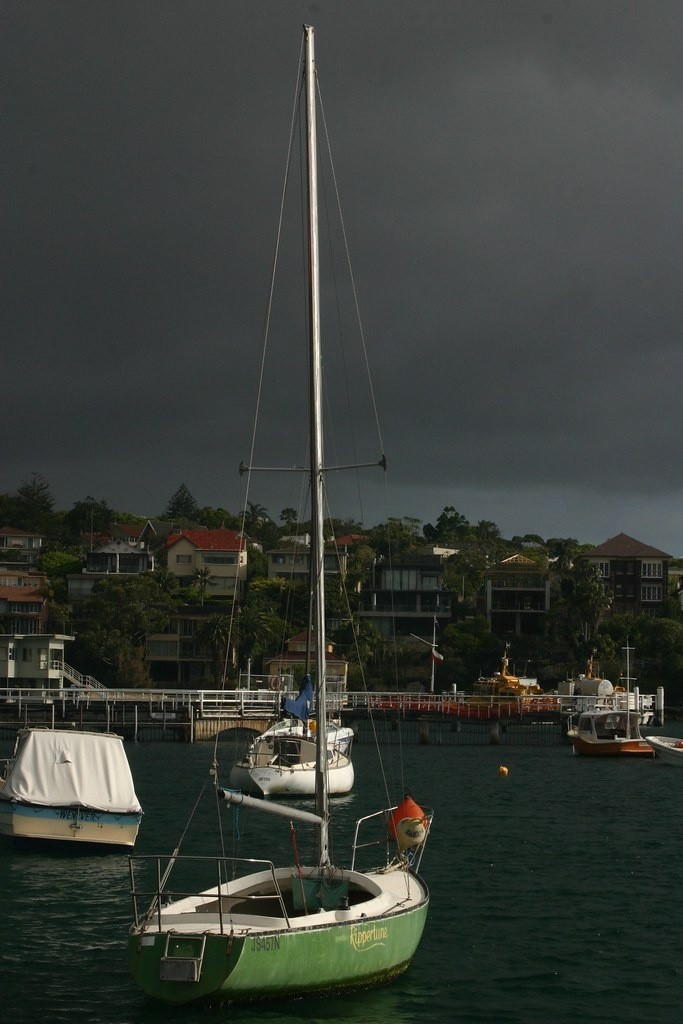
[643,734,683,768]
[0,727,144,848]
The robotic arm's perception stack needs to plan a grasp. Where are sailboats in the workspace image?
[567,634,656,758]
[113,20,624,1002]
